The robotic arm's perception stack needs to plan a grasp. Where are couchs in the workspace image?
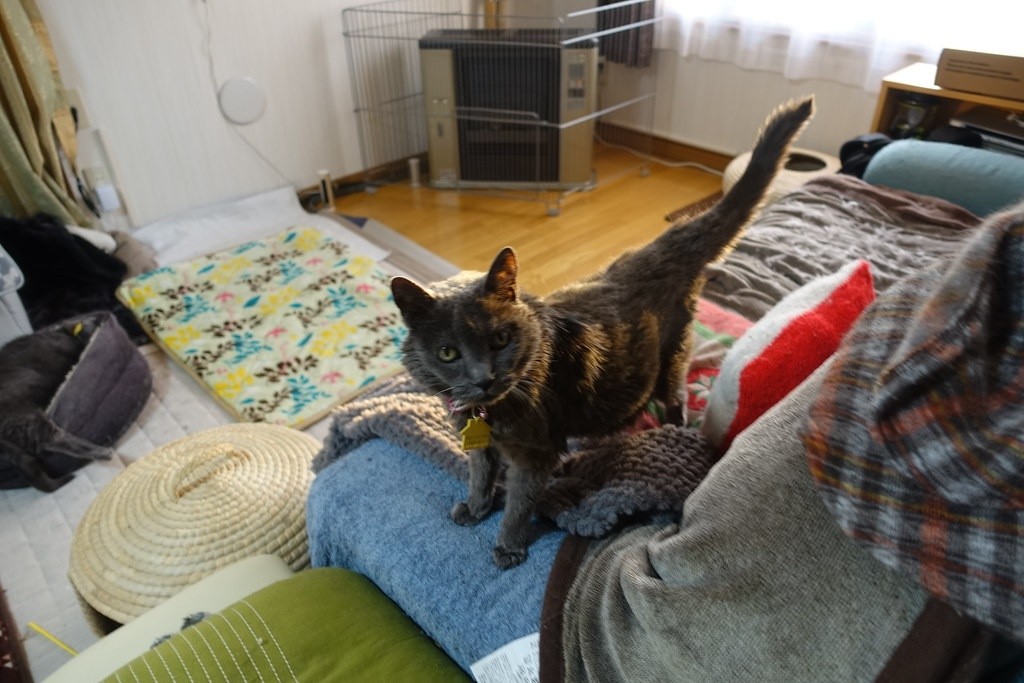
[2,140,1023,682]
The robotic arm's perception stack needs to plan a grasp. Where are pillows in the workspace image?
[703,259,875,456]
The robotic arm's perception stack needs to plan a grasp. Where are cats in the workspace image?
[0,314,114,493]
[390,93,815,571]
